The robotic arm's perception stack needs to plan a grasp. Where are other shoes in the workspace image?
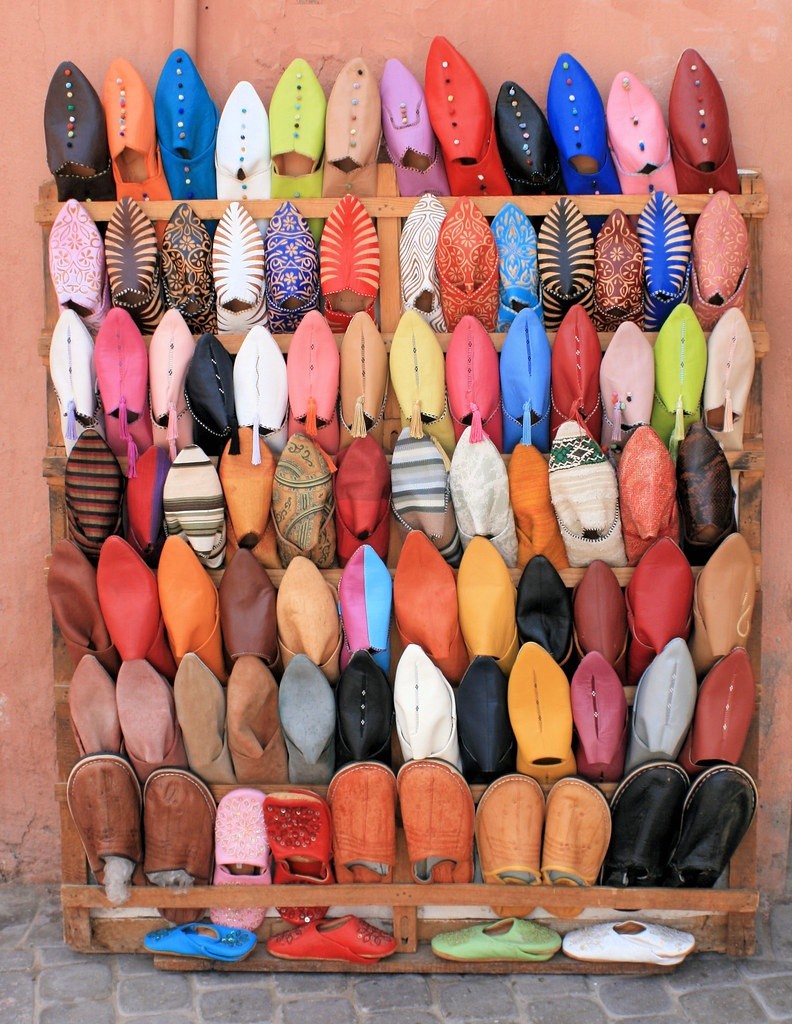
[431,918,561,963]
[45,35,782,769]
[563,920,696,965]
[542,781,609,917]
[329,760,398,884]
[398,760,475,885]
[603,760,691,911]
[666,767,758,887]
[267,914,397,964]
[476,772,546,917]
[143,922,257,962]
[214,789,269,930]
[65,756,143,889]
[263,788,334,924]
[141,766,211,924]
